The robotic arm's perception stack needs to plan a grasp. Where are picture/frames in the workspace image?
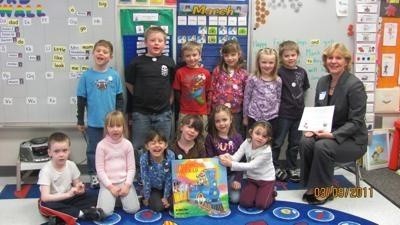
[365,129,391,171]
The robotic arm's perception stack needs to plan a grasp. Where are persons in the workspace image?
[37,132,104,225]
[208,40,244,135]
[94,110,140,217]
[139,104,277,212]
[297,43,368,205]
[129,25,176,186]
[273,40,310,182]
[77,40,126,188]
[173,40,212,156]
[242,47,283,134]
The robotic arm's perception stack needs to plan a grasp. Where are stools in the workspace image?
[329,158,361,198]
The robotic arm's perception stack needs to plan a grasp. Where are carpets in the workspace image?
[1,160,400,225]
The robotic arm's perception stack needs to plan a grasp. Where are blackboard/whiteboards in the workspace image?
[1,0,381,131]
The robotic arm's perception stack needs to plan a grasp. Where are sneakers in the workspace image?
[288,168,300,182]
[89,175,100,189]
[276,168,287,182]
[49,215,66,225]
[81,206,104,222]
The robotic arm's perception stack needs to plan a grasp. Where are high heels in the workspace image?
[303,186,336,205]
